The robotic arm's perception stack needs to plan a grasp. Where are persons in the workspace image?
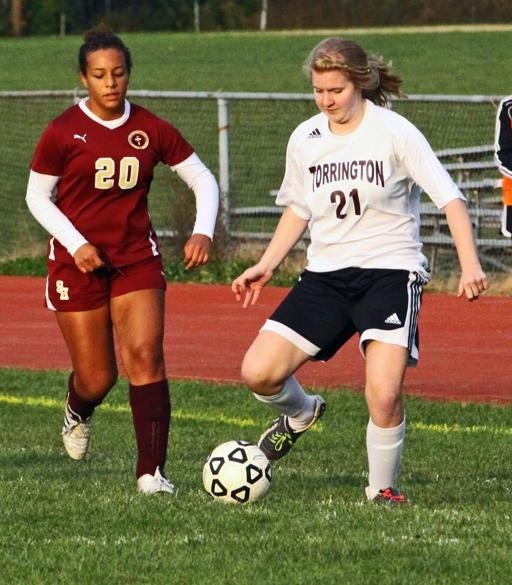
[493,94,512,242]
[228,31,491,514]
[18,20,221,502]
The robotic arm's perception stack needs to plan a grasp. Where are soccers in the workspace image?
[203,441,272,504]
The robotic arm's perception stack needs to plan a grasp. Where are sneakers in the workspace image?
[364,487,409,506]
[258,394,326,460]
[138,465,174,494]
[62,392,93,460]
